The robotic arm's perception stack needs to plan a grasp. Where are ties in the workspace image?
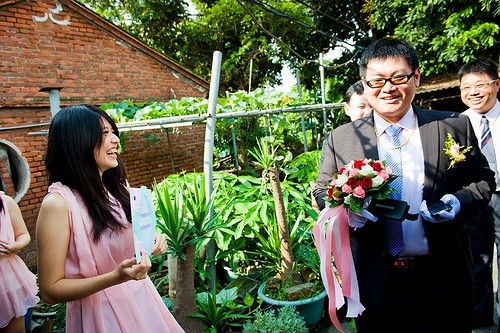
[384,126,405,256]
[480,116,499,186]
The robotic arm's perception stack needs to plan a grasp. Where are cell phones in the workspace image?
[427,200,454,217]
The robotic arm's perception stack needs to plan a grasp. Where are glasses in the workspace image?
[459,80,496,91]
[363,71,414,88]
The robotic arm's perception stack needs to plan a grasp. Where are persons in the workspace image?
[344,80,373,121]
[312,38,497,333]
[0,175,40,333]
[35,104,185,333]
[458,58,500,316]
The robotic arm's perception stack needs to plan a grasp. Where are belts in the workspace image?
[390,255,430,272]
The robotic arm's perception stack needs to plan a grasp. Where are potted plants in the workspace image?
[246,137,327,325]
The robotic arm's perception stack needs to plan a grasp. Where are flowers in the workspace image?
[443,134,473,171]
[323,158,398,227]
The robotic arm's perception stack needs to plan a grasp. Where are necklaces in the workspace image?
[374,113,416,149]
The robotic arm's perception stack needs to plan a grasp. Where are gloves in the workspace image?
[349,195,377,227]
[420,194,460,223]
